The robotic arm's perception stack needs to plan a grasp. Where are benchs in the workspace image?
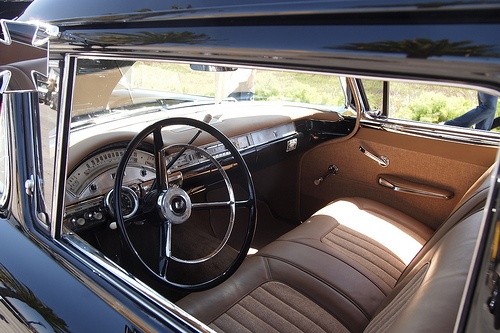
[172,152,500,333]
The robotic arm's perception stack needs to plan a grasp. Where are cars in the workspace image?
[1,0,500,333]
